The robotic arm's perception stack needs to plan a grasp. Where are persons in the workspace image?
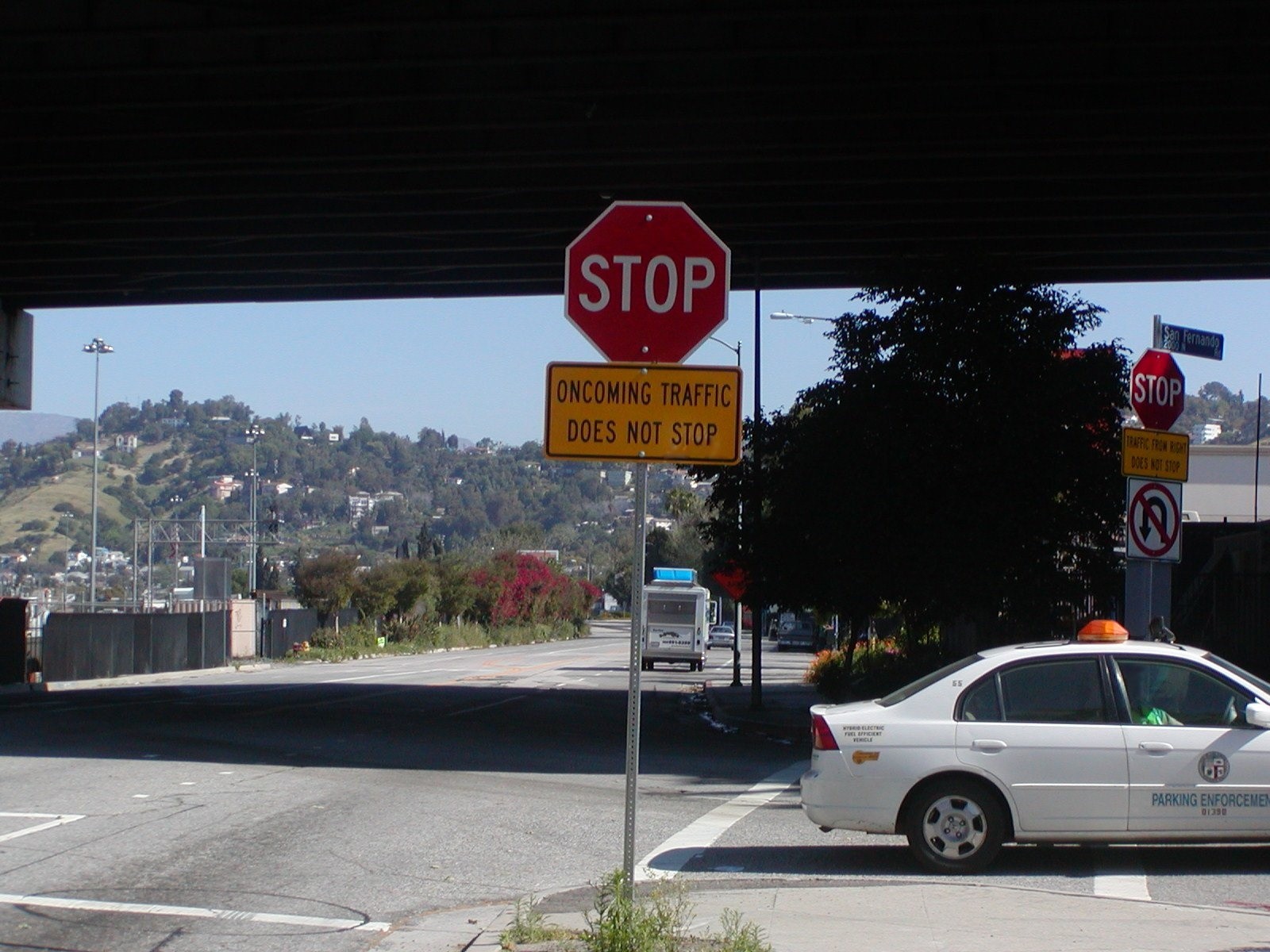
[1126,672,1184,726]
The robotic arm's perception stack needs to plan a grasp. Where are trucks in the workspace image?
[642,579,717,673]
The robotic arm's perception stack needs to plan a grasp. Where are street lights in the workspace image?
[243,468,260,598]
[79,335,115,613]
[243,424,266,594]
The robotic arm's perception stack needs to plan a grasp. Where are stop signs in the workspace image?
[562,198,733,362]
[1128,347,1186,433]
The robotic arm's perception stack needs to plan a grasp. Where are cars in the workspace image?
[774,620,818,653]
[722,621,735,631]
[799,618,1270,876]
[707,625,735,651]
